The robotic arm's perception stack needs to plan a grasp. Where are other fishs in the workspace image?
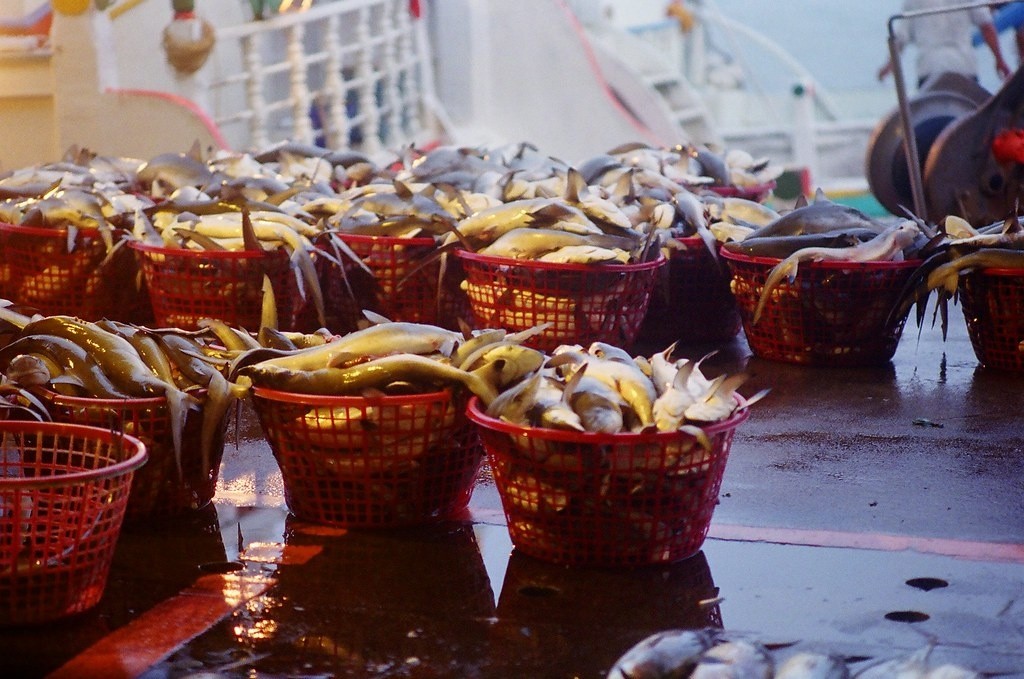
[1,138,1024,564]
[619,628,988,679]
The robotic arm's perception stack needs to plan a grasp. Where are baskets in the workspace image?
[249,384,488,531]
[467,389,752,567]
[957,265,1024,374]
[718,244,923,367]
[0,421,149,622]
[28,383,228,522]
[0,210,743,354]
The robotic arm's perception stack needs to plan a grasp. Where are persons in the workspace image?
[972,0,1024,65]
[876,0,1012,93]
